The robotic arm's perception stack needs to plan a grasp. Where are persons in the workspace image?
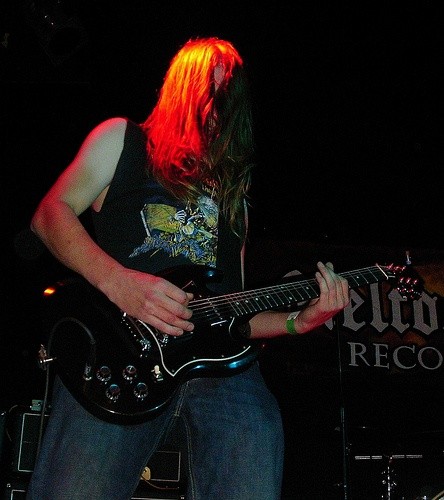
[26,37,351,500]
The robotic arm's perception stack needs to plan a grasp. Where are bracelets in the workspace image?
[284,309,300,338]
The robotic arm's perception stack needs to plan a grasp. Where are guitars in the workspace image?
[46,262,426,427]
